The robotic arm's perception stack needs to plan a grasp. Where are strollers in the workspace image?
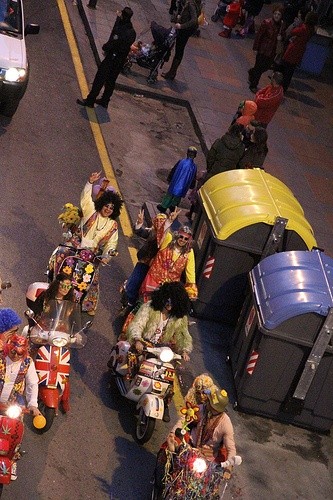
[211,0,234,23]
[120,20,180,85]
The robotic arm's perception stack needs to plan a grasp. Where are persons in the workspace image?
[49,170,123,330]
[77,7,137,107]
[169,0,203,37]
[249,5,284,95]
[279,0,320,94]
[0,334,43,481]
[120,208,168,306]
[162,0,199,80]
[253,72,284,128]
[28,272,86,344]
[162,375,238,485]
[131,40,157,58]
[142,206,199,317]
[186,97,269,220]
[212,0,263,39]
[157,145,198,213]
[126,280,194,424]
[87,0,98,9]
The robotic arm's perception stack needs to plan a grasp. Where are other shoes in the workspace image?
[161,72,174,81]
[164,407,171,422]
[95,98,108,108]
[219,32,229,38]
[169,206,176,212]
[157,204,167,213]
[76,98,95,108]
[251,87,258,94]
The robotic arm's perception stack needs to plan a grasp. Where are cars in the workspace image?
[0,0,40,119]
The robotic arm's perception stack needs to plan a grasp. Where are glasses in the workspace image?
[60,284,72,289]
[179,234,190,240]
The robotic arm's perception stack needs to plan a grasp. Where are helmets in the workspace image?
[207,391,229,413]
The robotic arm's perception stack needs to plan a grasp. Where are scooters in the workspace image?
[116,279,137,321]
[153,427,229,500]
[106,336,185,445]
[0,403,34,498]
[43,221,119,313]
[18,299,93,435]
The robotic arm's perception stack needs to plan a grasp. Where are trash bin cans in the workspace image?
[190,171,318,327]
[225,249,333,435]
